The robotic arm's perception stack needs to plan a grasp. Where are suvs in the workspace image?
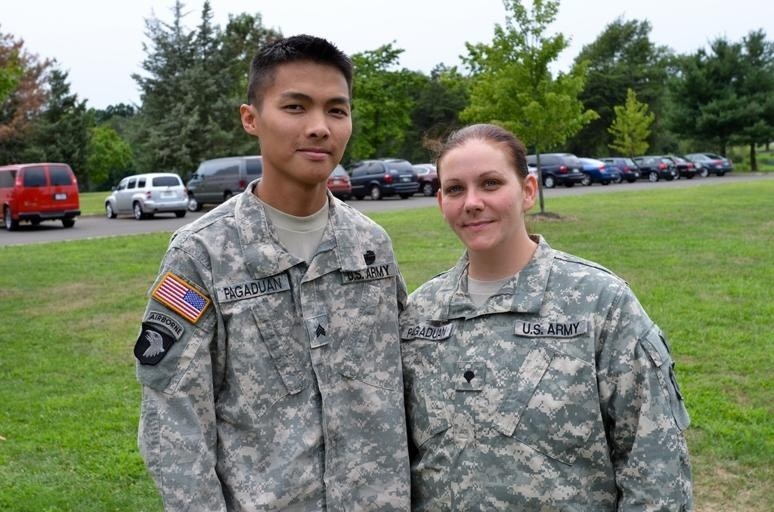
[327,155,440,202]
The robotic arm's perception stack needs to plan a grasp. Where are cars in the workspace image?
[101,171,190,222]
[525,150,734,189]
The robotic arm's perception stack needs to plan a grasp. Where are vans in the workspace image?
[186,154,266,212]
[1,160,83,233]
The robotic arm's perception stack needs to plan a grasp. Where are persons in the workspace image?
[396,123,696,511]
[133,31,424,511]
